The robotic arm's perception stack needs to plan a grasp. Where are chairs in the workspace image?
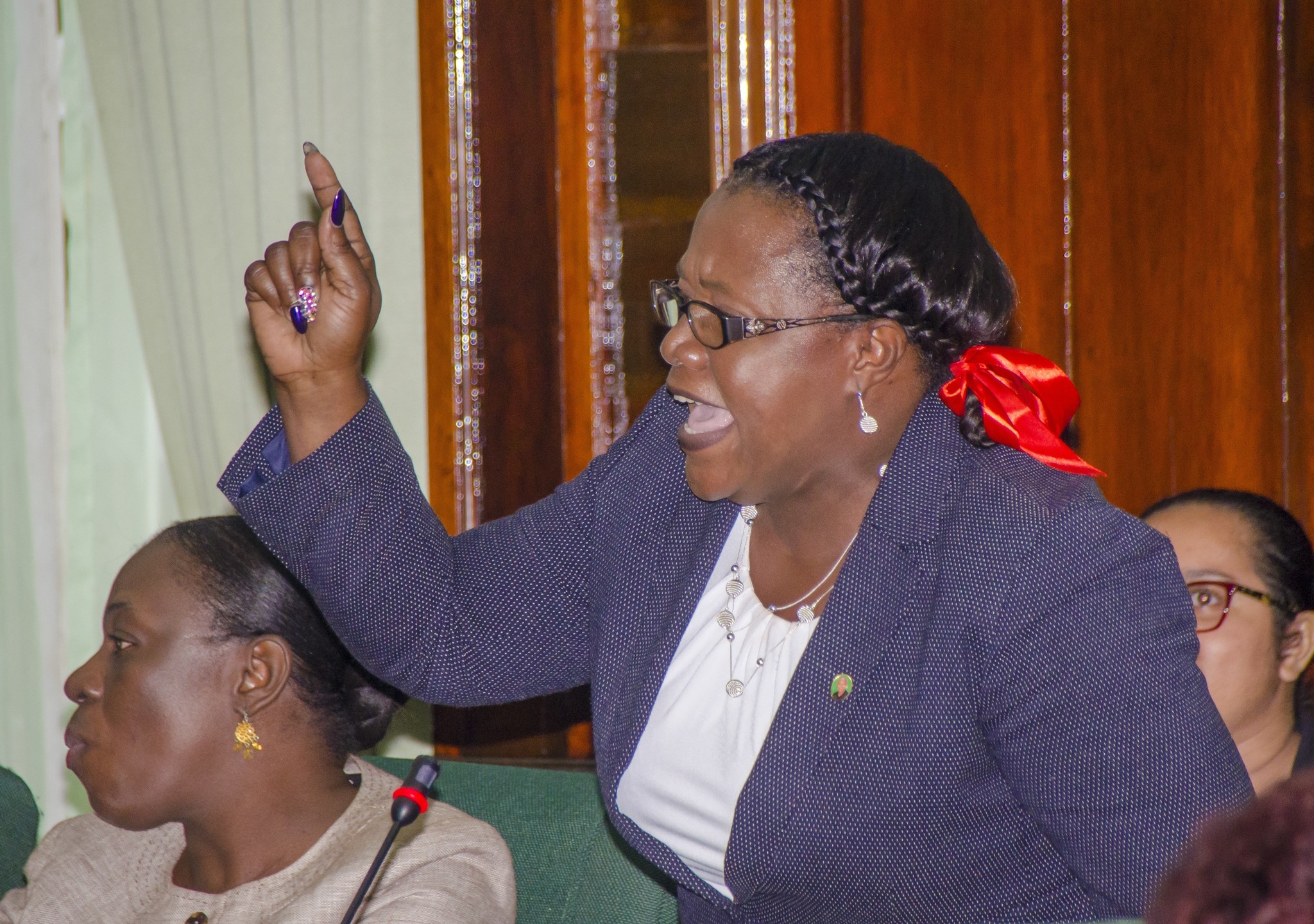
[359,754,679,924]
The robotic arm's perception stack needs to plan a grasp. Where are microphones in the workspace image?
[340,753,442,923]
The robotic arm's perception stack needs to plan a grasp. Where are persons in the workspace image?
[216,134,1256,923]
[1,515,517,924]
[1140,486,1314,795]
[1146,768,1314,924]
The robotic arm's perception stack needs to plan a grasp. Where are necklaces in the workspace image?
[716,464,889,697]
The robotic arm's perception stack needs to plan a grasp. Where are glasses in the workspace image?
[648,279,915,351]
[1184,581,1300,633]
